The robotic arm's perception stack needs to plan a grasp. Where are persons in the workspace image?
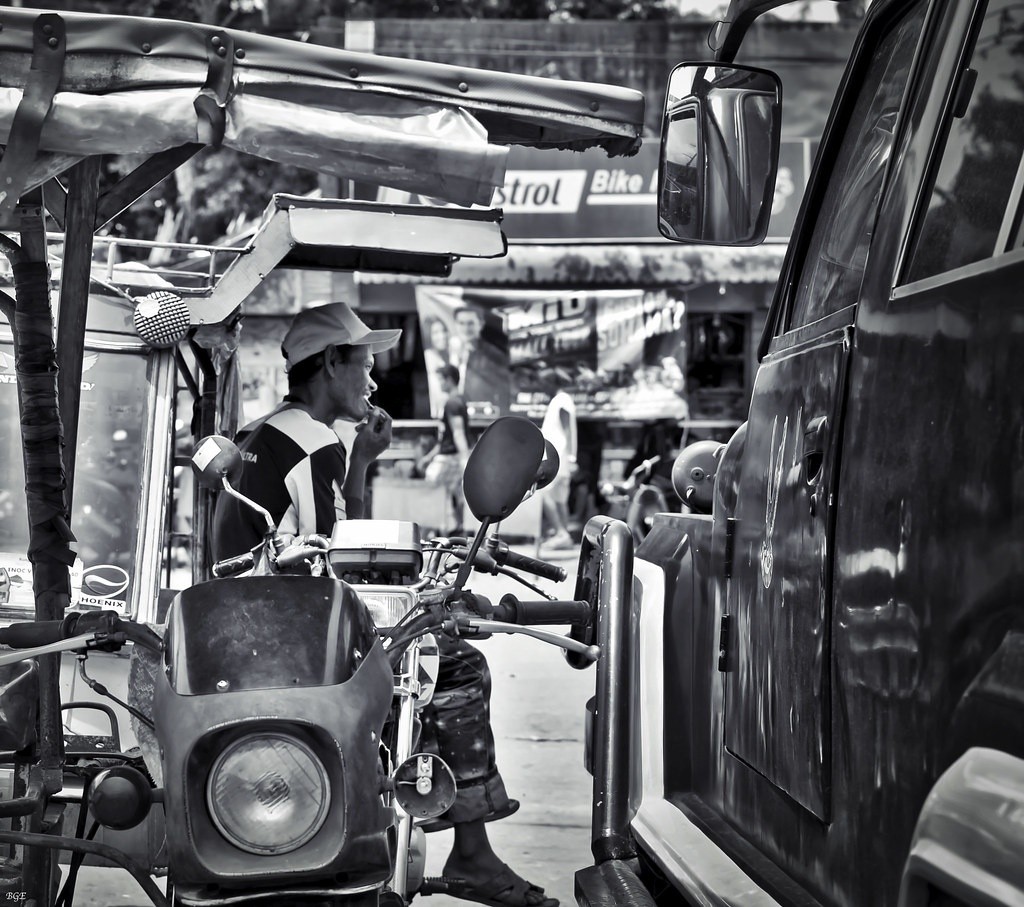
[416,366,474,538]
[537,368,578,550]
[208,300,558,907]
[421,307,511,420]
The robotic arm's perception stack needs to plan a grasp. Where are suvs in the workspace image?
[562,1,1024,907]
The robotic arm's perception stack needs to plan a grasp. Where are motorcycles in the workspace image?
[0,409,604,907]
[210,442,569,907]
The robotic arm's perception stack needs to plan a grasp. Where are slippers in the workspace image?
[415,799,520,832]
[445,863,559,907]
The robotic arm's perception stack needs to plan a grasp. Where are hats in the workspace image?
[283,303,402,373]
